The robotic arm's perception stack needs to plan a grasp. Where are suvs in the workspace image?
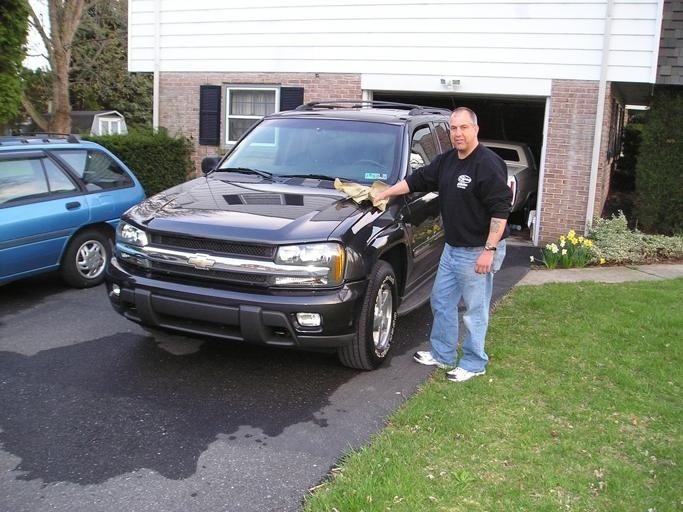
[105,99,474,371]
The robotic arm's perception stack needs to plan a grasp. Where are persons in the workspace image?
[372,106,513,382]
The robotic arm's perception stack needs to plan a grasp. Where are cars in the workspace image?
[476,139,538,225]
[0,133,148,288]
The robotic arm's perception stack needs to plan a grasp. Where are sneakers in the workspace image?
[446,367,486,384]
[413,350,454,371]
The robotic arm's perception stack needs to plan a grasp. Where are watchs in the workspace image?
[483,243,497,251]
